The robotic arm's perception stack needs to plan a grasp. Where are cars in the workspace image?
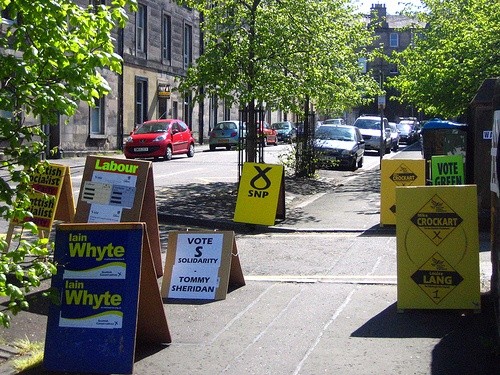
[257,121,278,147]
[390,124,402,151]
[123,119,195,160]
[293,118,345,142]
[353,114,393,157]
[314,125,365,172]
[271,122,297,145]
[208,120,249,151]
[396,120,420,146]
